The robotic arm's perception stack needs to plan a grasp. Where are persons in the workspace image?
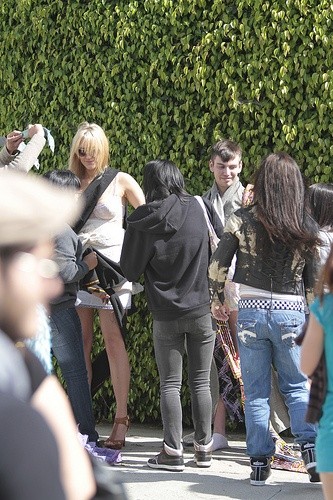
[1,114,332,500]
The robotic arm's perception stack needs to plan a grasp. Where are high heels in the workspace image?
[104,415,130,449]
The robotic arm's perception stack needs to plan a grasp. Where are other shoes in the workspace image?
[249,456,274,486]
[184,432,195,446]
[300,442,321,482]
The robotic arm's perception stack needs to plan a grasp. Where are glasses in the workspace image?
[77,147,87,157]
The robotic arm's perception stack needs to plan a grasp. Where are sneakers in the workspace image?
[193,451,213,467]
[147,448,186,471]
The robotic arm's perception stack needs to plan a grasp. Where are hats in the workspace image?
[0,169,83,248]
